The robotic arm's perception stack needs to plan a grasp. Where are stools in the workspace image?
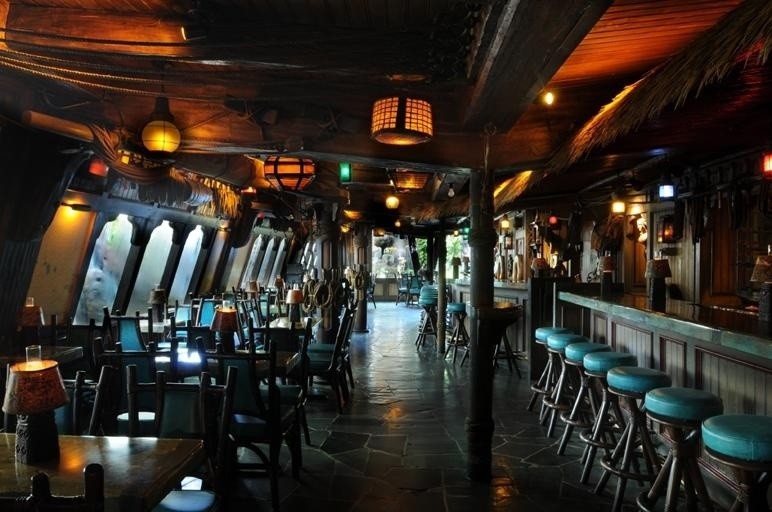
[419,285,527,379]
[526,325,670,512]
[636,384,722,512]
[697,413,772,512]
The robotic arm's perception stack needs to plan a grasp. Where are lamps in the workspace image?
[751,256,772,321]
[140,60,180,164]
[531,258,547,278]
[447,184,454,197]
[598,255,617,300]
[644,259,672,311]
[89,158,109,177]
[264,148,316,194]
[500,212,510,228]
[369,91,433,145]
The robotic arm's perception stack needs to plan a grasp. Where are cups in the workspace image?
[26,345,42,369]
[652,247,662,261]
[605,250,611,256]
[26,296,34,308]
[154,274,299,309]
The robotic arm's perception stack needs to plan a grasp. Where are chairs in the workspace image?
[393,273,423,307]
